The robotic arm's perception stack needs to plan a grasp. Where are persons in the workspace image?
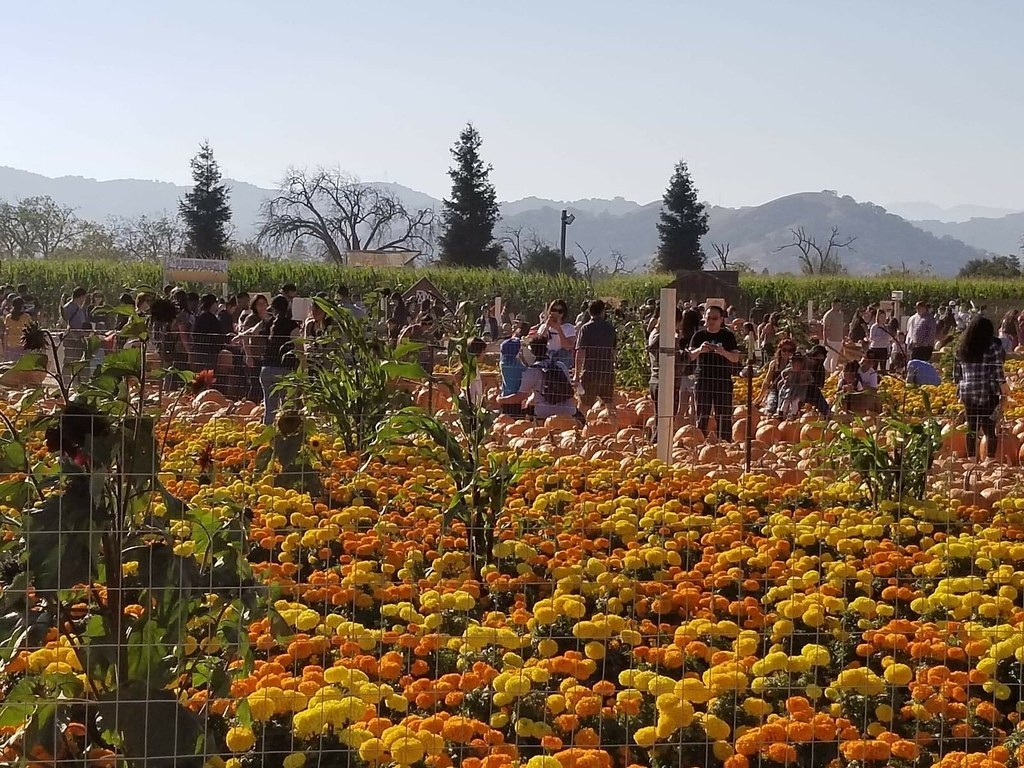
[0,272,1024,461]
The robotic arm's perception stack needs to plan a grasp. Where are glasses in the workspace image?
[551,308,564,314]
[781,347,795,352]
[287,294,299,299]
[705,316,719,321]
[144,299,151,302]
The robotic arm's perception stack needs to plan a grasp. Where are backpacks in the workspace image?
[163,325,179,360]
[529,359,575,405]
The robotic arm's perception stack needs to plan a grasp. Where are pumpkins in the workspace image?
[0,385,1024,515]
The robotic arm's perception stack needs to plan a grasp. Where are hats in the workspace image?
[913,301,926,308]
[949,301,956,305]
[755,298,763,304]
